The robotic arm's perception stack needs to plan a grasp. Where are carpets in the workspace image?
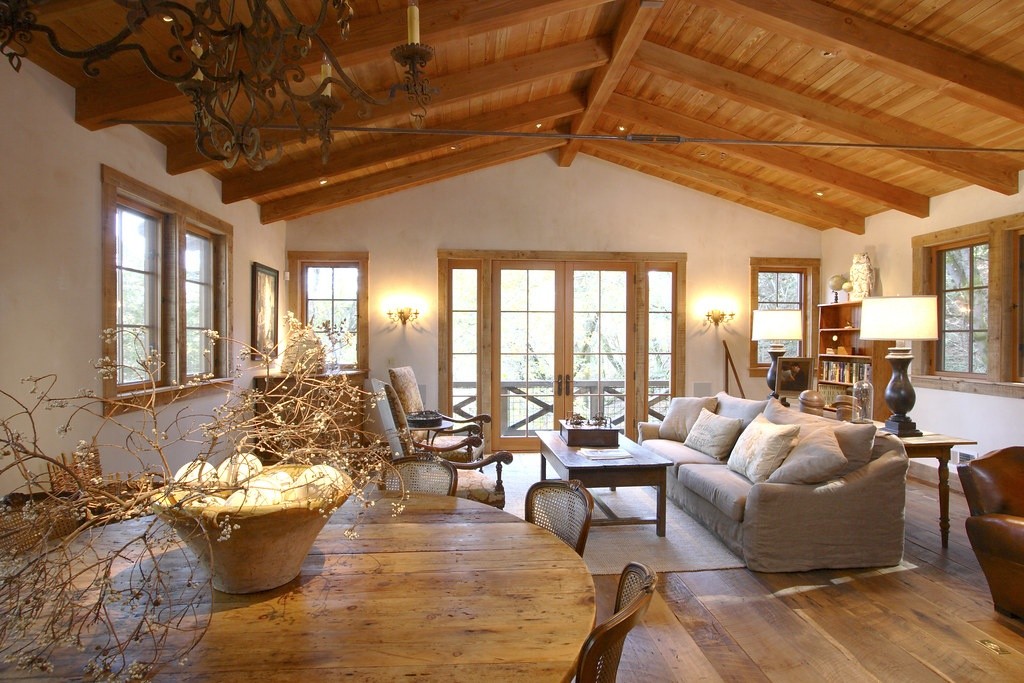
[481,453,748,574]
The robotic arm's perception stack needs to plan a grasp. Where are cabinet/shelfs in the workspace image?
[817,302,896,424]
[255,371,365,464]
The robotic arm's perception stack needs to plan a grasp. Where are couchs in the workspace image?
[637,390,909,573]
[955,445,1024,618]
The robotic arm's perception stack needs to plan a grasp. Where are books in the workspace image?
[817,383,847,407]
[820,361,872,384]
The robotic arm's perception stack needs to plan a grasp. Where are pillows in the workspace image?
[684,408,744,461]
[659,396,718,442]
[769,425,848,484]
[727,412,800,484]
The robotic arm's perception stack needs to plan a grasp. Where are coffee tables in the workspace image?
[535,430,675,538]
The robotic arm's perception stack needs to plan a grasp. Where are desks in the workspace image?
[0,494,598,683]
[864,418,978,548]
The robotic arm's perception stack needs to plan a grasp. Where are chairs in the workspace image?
[385,383,514,509]
[524,476,593,556]
[377,454,458,497]
[387,366,493,472]
[577,561,659,683]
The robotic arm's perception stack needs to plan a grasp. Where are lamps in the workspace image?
[0,0,436,171]
[751,309,803,406]
[705,308,736,326]
[859,296,938,436]
[388,307,419,324]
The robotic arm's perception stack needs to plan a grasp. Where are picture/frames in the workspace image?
[776,357,815,395]
[252,260,280,361]
